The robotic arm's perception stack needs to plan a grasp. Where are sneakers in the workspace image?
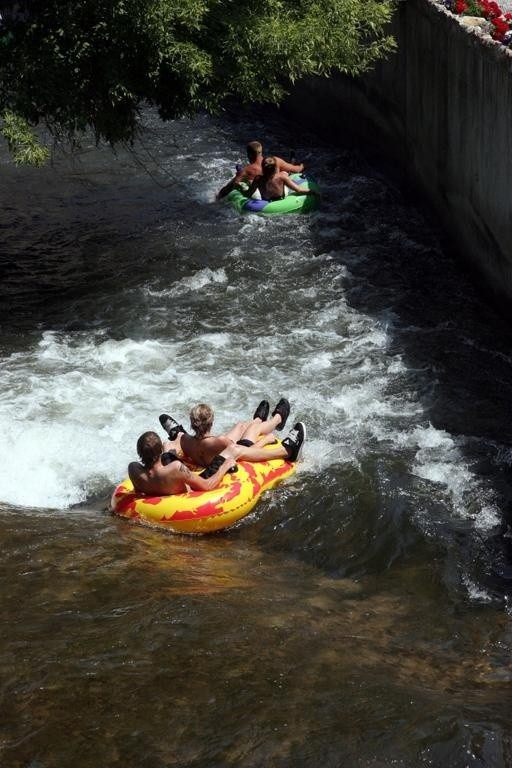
[159,414,186,439]
[253,399,269,421]
[281,422,307,462]
[272,398,290,431]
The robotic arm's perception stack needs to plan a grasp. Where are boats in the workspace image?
[222,174,316,218]
[110,434,296,534]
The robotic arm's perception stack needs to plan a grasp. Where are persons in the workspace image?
[128,414,306,497]
[233,158,320,202]
[180,397,290,468]
[215,141,305,202]
